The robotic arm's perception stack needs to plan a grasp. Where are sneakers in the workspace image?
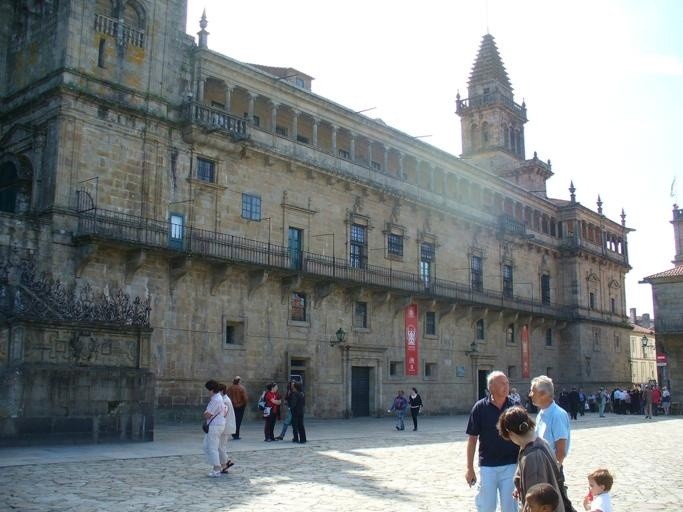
[396,426,417,431]
[266,435,307,443]
[208,459,233,477]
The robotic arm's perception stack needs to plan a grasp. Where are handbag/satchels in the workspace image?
[203,421,210,433]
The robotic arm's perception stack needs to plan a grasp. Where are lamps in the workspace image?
[331,328,345,347]
[642,335,656,347]
[467,341,477,356]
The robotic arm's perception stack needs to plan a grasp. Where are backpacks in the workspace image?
[258,391,267,410]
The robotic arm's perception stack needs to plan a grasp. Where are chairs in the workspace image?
[670,401,681,414]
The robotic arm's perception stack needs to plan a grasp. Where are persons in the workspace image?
[226,375,247,440]
[527,375,571,498]
[582,468,613,512]
[524,482,559,512]
[203,379,226,477]
[510,382,671,420]
[217,383,236,473]
[289,381,306,443]
[263,381,282,442]
[274,378,296,440]
[464,370,525,511]
[496,404,575,512]
[385,388,408,431]
[407,386,423,432]
